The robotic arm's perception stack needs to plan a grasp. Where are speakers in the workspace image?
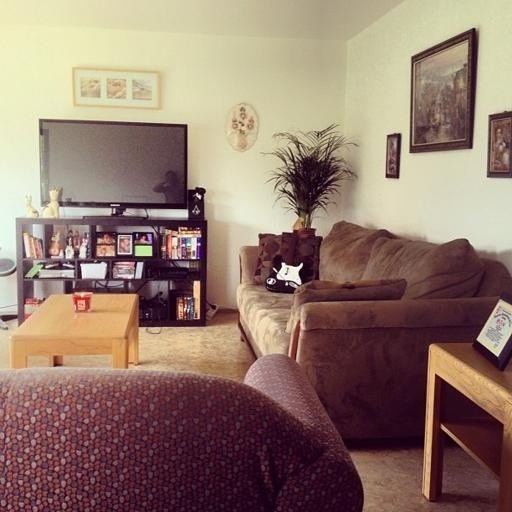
[190,189,205,223]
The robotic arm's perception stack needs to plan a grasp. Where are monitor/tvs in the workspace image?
[39,119,188,222]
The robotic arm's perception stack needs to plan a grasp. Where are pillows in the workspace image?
[252,229,295,289]
[283,278,408,335]
[265,255,309,296]
[282,232,323,283]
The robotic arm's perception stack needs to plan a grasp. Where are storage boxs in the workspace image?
[80,262,109,281]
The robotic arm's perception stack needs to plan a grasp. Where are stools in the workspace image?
[0,256,18,333]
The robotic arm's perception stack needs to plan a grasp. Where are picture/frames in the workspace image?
[485,110,512,180]
[133,230,154,257]
[93,230,117,259]
[70,64,162,112]
[406,27,477,156]
[385,132,401,179]
[472,291,511,372]
[116,232,133,257]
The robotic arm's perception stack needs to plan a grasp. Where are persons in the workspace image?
[120,238,129,253]
[134,234,150,245]
[103,233,112,244]
[98,249,110,256]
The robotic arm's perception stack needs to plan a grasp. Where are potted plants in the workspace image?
[258,123,360,238]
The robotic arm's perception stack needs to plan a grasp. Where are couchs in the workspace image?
[233,219,512,445]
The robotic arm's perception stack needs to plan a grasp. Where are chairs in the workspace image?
[1,350,368,512]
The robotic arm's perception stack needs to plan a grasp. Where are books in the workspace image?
[158,226,203,262]
[176,279,201,321]
[22,232,42,260]
[112,262,135,279]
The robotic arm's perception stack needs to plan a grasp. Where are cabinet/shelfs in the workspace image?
[418,340,512,511]
[13,216,209,329]
